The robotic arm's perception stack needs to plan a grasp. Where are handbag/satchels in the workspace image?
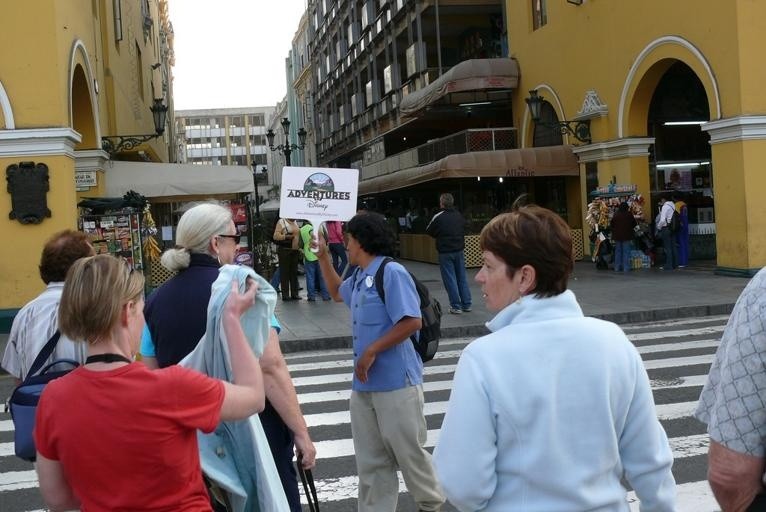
[3,316,81,463]
[272,218,293,249]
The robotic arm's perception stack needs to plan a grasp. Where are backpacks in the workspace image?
[664,203,681,234]
[373,257,443,364]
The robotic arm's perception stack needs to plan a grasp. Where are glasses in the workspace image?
[216,234,242,244]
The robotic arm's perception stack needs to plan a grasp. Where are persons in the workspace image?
[425,192,473,314]
[431,201,678,512]
[692,264,766,512]
[144,201,316,512]
[267,208,368,302]
[308,209,447,512]
[654,194,676,270]
[31,253,267,512]
[610,202,637,273]
[1,228,136,466]
[672,189,690,267]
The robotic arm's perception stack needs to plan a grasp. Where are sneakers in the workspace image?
[463,307,472,312]
[447,307,463,315]
[277,287,332,302]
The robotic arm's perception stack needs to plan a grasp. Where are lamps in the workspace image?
[523,89,590,144]
[102,93,167,157]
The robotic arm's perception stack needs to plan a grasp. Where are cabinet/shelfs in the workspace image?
[78,211,153,303]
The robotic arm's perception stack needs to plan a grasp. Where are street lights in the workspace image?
[250,159,267,220]
[266,119,308,168]
